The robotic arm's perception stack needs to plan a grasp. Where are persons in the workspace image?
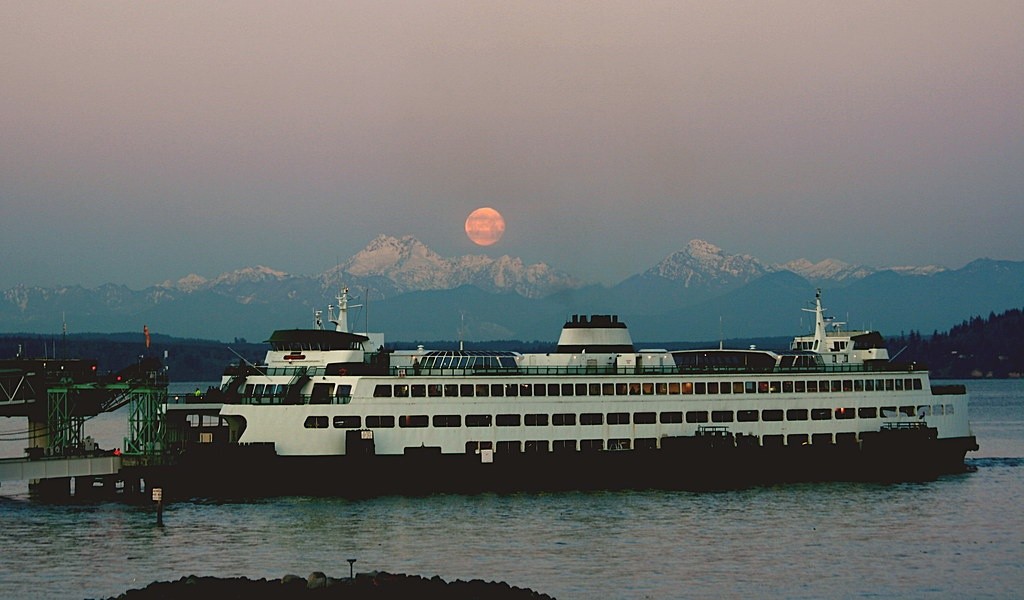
[194,387,200,397]
[412,358,420,376]
[113,448,120,457]
[207,386,221,398]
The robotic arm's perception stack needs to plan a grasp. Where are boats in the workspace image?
[155,288,981,497]
[0,343,174,418]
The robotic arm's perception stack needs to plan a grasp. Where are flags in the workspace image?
[144,326,151,348]
